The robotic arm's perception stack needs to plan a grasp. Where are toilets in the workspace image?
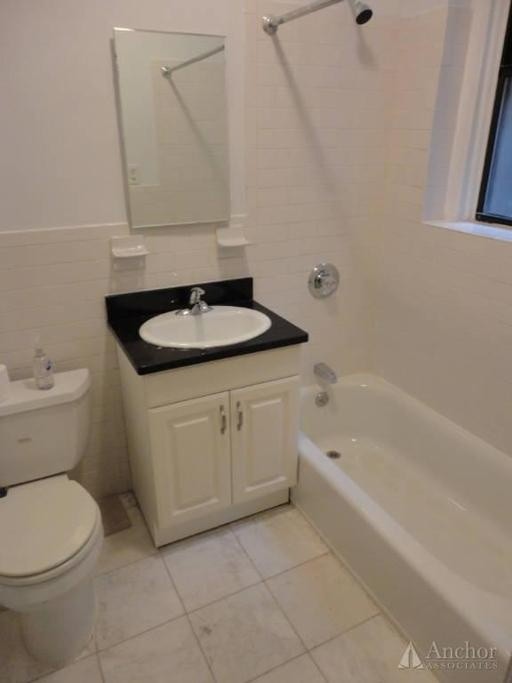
[0,367,102,668]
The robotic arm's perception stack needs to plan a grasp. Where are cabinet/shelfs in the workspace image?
[103,281,308,549]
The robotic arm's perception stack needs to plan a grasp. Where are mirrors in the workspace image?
[108,25,235,228]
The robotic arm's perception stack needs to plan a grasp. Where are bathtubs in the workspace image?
[289,372,512,683]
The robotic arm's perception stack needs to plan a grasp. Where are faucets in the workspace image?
[313,362,338,385]
[189,287,212,315]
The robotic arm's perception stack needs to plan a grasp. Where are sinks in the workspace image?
[138,305,272,350]
